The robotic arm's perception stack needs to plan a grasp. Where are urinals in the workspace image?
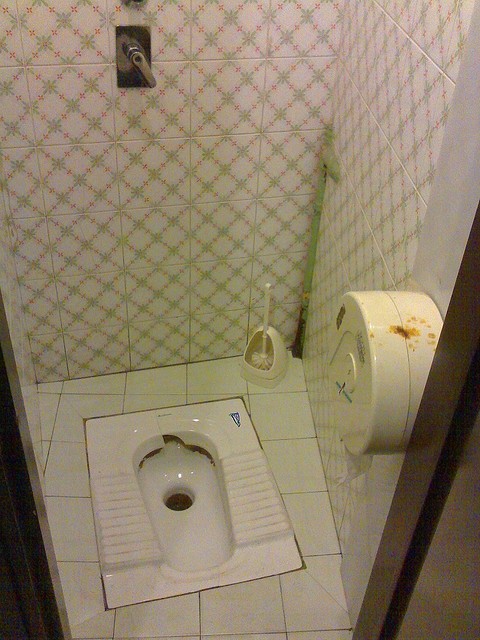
[85,398,306,609]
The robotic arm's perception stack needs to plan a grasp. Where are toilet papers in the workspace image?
[325,290,443,487]
